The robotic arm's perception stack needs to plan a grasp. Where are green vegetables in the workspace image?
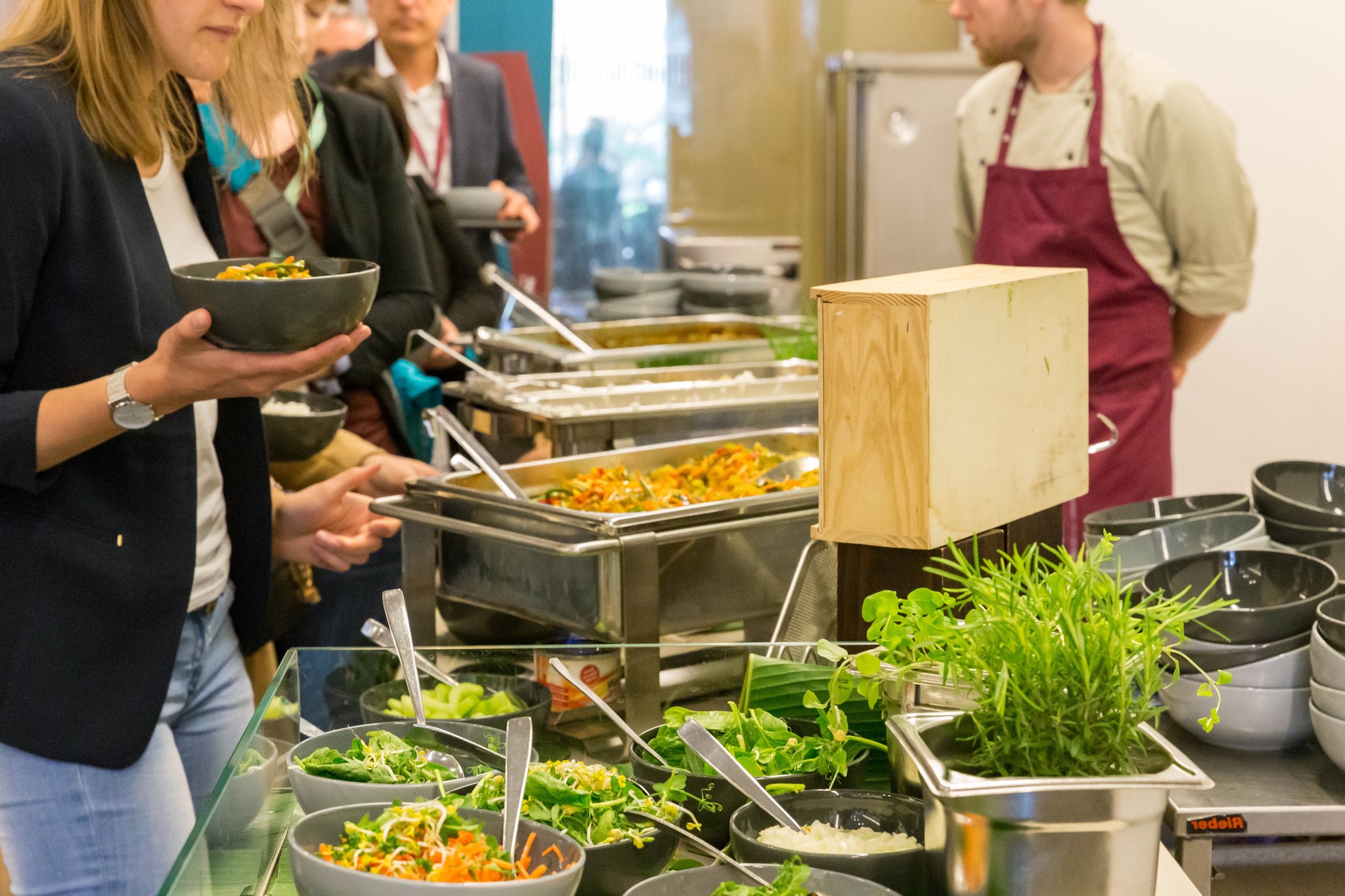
[289,699,888,896]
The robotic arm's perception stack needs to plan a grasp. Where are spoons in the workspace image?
[380,589,466,779]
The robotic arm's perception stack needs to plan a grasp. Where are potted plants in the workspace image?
[877,522,1241,896]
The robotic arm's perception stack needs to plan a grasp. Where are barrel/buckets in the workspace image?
[533,637,620,711]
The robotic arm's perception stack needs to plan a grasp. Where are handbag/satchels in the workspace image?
[387,358,445,469]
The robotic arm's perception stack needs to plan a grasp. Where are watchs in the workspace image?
[106,361,164,429]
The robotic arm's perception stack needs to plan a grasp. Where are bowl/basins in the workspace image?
[435,590,568,646]
[284,672,937,896]
[586,231,800,321]
[204,733,278,842]
[259,390,348,462]
[1082,461,1345,777]
[168,256,381,354]
[439,187,503,220]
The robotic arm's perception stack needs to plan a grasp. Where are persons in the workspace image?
[950,0,1257,561]
[556,124,622,292]
[0,0,404,896]
[186,0,543,739]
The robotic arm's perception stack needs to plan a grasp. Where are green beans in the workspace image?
[380,681,519,719]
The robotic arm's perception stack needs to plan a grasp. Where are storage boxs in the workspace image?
[808,263,1093,550]
